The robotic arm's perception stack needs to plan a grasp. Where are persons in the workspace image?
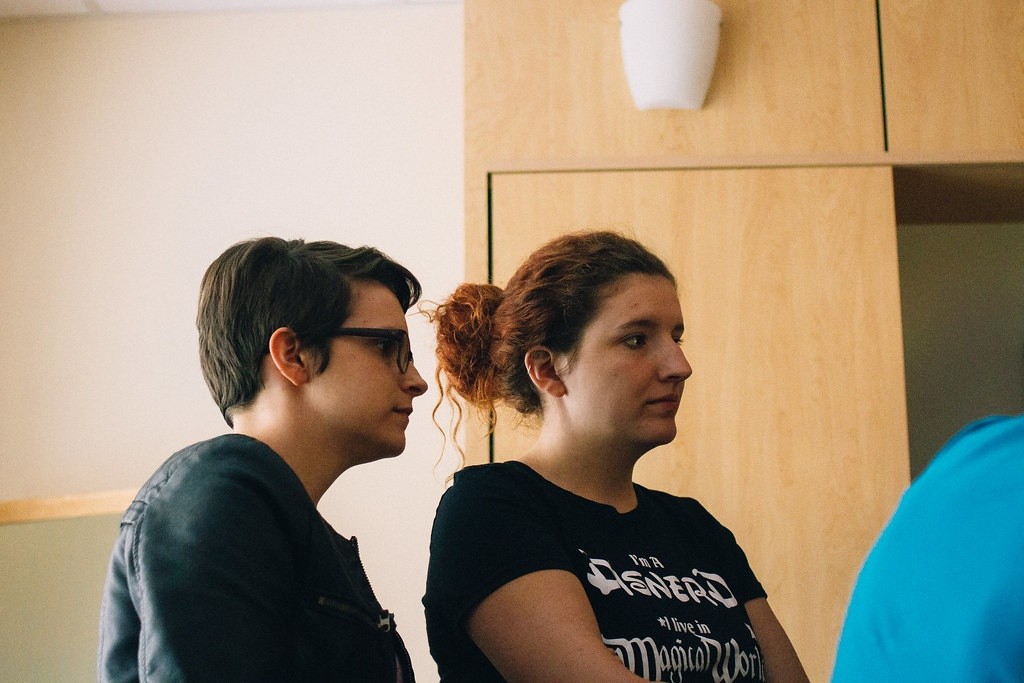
[423,230,811,683]
[97,236,429,683]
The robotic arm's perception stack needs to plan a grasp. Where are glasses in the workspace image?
[330,328,414,374]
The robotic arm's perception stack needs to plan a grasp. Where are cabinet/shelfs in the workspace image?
[464,0,1024,683]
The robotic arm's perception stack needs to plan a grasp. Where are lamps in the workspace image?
[620,0,724,113]
